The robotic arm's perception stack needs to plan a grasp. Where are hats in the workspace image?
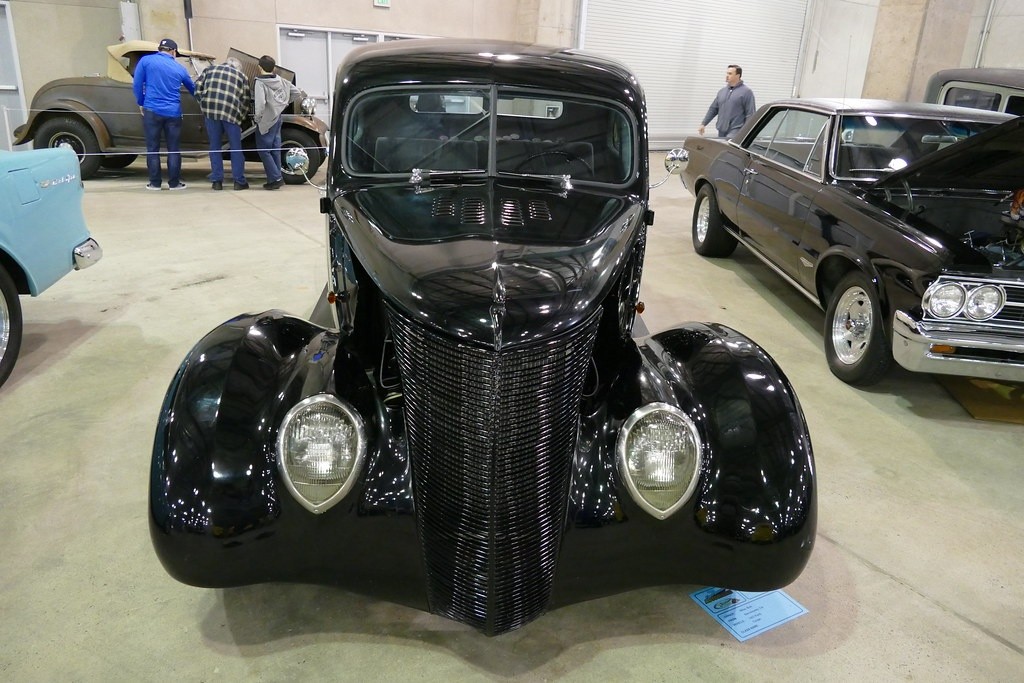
[160,39,181,57]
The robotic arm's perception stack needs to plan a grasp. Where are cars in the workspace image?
[11,42,332,185]
[145,37,821,638]
[671,88,1023,386]
[922,69,1024,118]
[1,145,101,389]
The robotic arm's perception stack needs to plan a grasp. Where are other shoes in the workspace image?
[212,181,223,190]
[263,180,283,190]
[233,182,249,190]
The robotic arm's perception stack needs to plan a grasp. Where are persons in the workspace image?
[194,57,251,190]
[413,94,446,113]
[252,55,301,190]
[133,39,195,190]
[698,65,756,138]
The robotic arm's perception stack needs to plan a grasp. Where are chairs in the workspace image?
[494,137,595,181]
[838,142,895,182]
[373,137,480,173]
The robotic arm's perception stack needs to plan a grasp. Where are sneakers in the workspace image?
[169,183,187,191]
[146,182,162,190]
